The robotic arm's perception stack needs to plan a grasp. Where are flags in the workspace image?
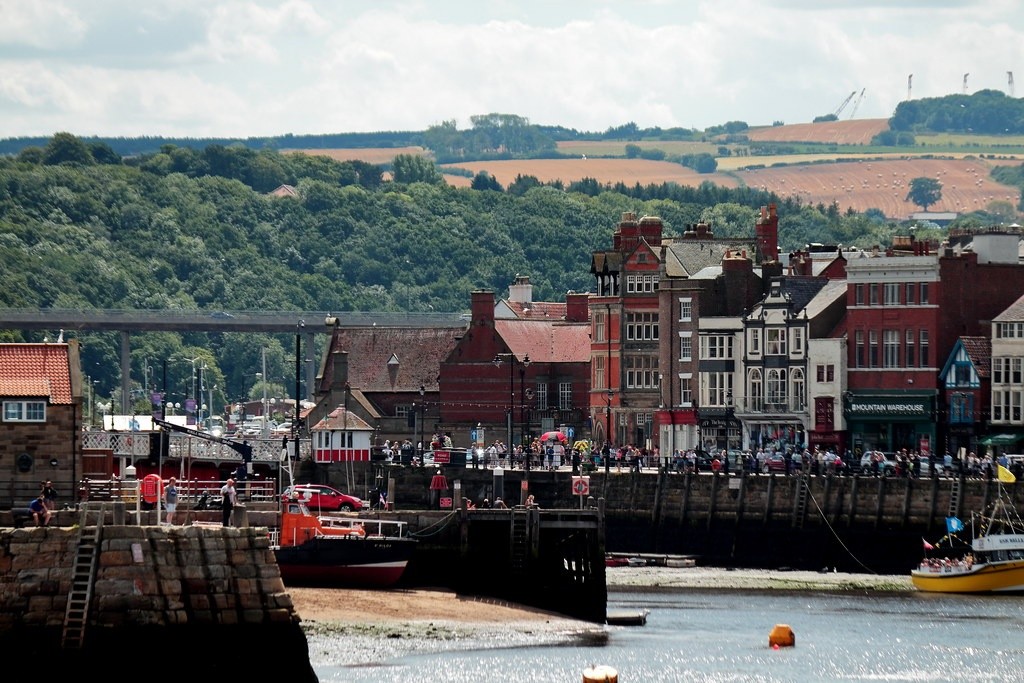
[945,516,964,532]
[998,464,1017,483]
[923,539,934,550]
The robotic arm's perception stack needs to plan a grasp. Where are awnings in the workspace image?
[698,414,739,428]
[980,432,1023,445]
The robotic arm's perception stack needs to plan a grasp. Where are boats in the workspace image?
[910,462,1024,592]
[261,496,421,589]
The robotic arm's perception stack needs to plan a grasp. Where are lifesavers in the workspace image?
[573,480,588,494]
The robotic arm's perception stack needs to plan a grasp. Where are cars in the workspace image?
[205,416,293,440]
[282,485,364,512]
[861,449,899,475]
[908,456,946,476]
[467,449,483,463]
[762,454,788,473]
[423,452,438,464]
[723,448,745,471]
[669,450,717,470]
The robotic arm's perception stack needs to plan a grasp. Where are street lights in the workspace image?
[97,402,112,431]
[166,401,181,417]
[520,368,535,472]
[194,404,208,426]
[509,353,530,468]
[419,384,426,466]
[261,398,276,421]
[92,380,101,427]
[294,319,306,419]
[162,358,177,421]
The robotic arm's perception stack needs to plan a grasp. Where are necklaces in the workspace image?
[464,432,555,510]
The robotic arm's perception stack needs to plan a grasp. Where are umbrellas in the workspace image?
[540,431,566,443]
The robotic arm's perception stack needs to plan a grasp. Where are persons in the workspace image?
[958,445,1018,481]
[921,551,974,574]
[162,477,179,528]
[286,491,300,513]
[371,435,450,512]
[559,435,961,482]
[220,478,237,527]
[30,480,57,528]
